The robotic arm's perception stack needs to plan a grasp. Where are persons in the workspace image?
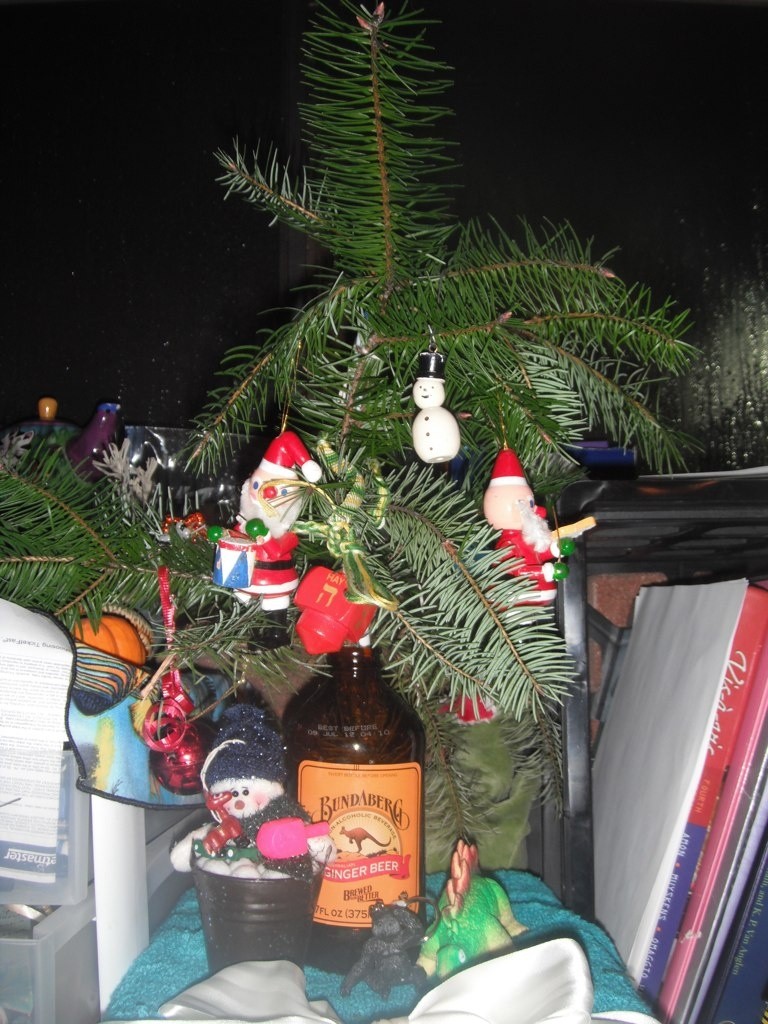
[208,432,322,610]
[484,448,574,611]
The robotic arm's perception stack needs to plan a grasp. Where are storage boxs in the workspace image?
[0,746,208,1024]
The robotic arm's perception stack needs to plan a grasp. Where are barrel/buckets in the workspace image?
[190,853,310,976]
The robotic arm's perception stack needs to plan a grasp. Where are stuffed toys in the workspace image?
[170,705,338,882]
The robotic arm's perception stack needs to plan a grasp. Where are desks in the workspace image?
[100,865,660,1024]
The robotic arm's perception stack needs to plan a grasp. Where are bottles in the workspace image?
[277,646,424,975]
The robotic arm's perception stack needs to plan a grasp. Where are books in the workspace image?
[592,578,768,1024]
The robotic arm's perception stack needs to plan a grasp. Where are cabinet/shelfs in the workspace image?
[530,479,768,1024]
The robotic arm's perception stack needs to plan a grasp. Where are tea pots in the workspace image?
[1,397,120,492]
